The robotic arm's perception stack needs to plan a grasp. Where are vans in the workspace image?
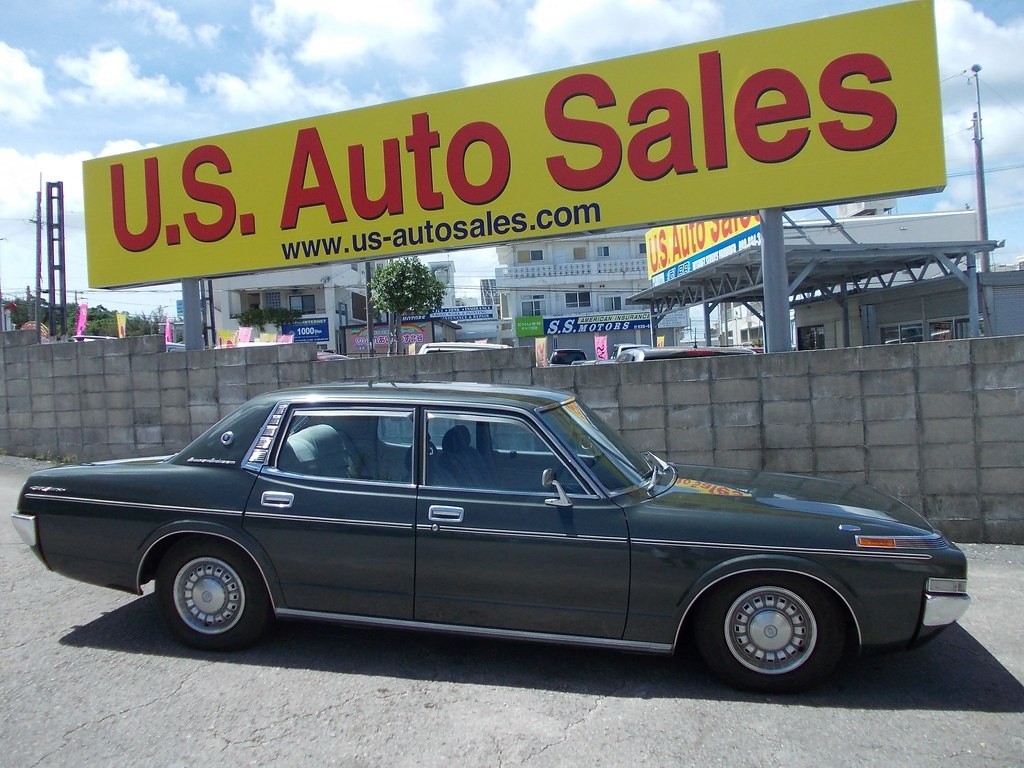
[418,341,511,355]
[613,344,652,361]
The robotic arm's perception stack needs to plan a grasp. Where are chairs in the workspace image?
[285,423,357,478]
[433,424,491,490]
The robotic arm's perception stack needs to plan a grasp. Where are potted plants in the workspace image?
[235,307,306,342]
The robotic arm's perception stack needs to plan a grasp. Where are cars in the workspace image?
[10,376,976,697]
[619,346,758,361]
[550,348,588,365]
